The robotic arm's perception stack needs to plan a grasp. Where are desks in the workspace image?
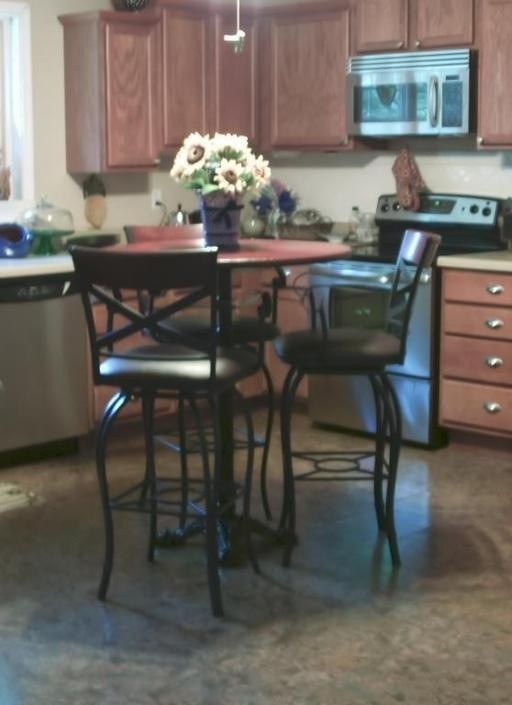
[104,238,351,560]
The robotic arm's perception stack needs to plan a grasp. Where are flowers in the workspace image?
[169,131,271,206]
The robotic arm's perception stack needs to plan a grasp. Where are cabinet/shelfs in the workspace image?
[153,0,256,158]
[436,247,512,440]
[474,0,512,151]
[255,0,355,152]
[350,0,479,53]
[57,10,158,174]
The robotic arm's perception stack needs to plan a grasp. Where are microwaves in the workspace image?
[346,47,477,142]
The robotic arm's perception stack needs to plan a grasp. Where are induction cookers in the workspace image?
[349,240,499,266]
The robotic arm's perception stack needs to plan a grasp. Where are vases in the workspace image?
[201,205,245,253]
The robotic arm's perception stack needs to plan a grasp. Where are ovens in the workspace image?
[309,259,447,448]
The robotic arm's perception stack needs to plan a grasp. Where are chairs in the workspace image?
[124,223,277,563]
[269,230,442,568]
[66,243,260,618]
